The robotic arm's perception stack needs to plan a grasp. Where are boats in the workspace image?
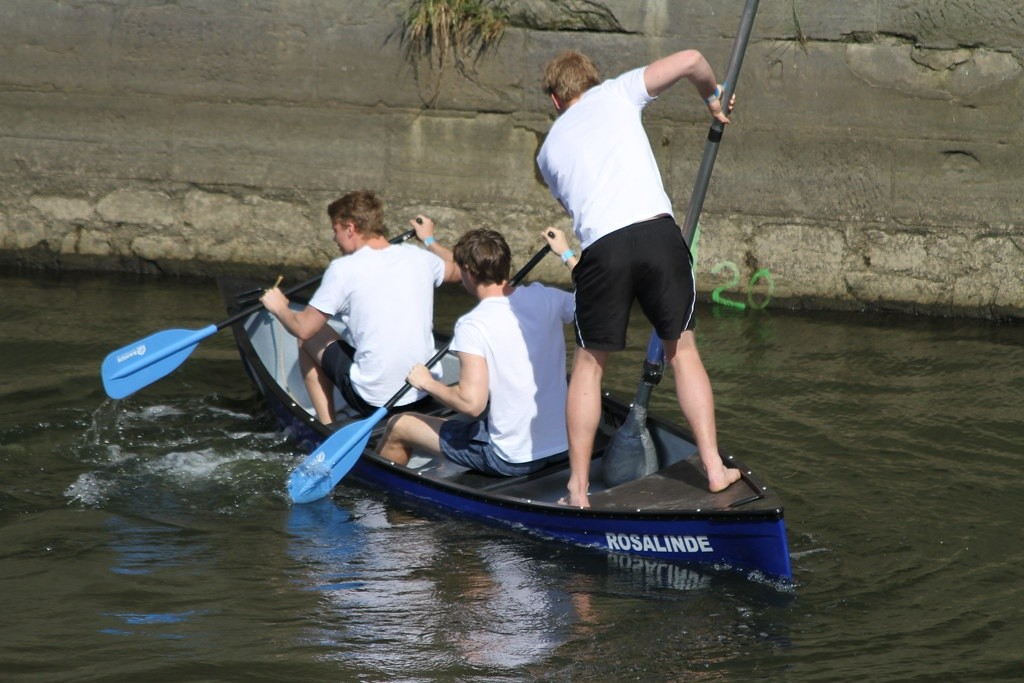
[224,284,793,586]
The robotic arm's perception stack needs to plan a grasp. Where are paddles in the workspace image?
[284,229,552,507]
[596,0,760,489]
[98,217,424,401]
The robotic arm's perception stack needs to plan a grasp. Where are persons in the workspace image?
[258,190,461,424]
[537,48,740,505]
[374,228,578,474]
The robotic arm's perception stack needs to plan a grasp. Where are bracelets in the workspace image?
[561,250,575,265]
[707,89,719,103]
[424,237,436,246]
